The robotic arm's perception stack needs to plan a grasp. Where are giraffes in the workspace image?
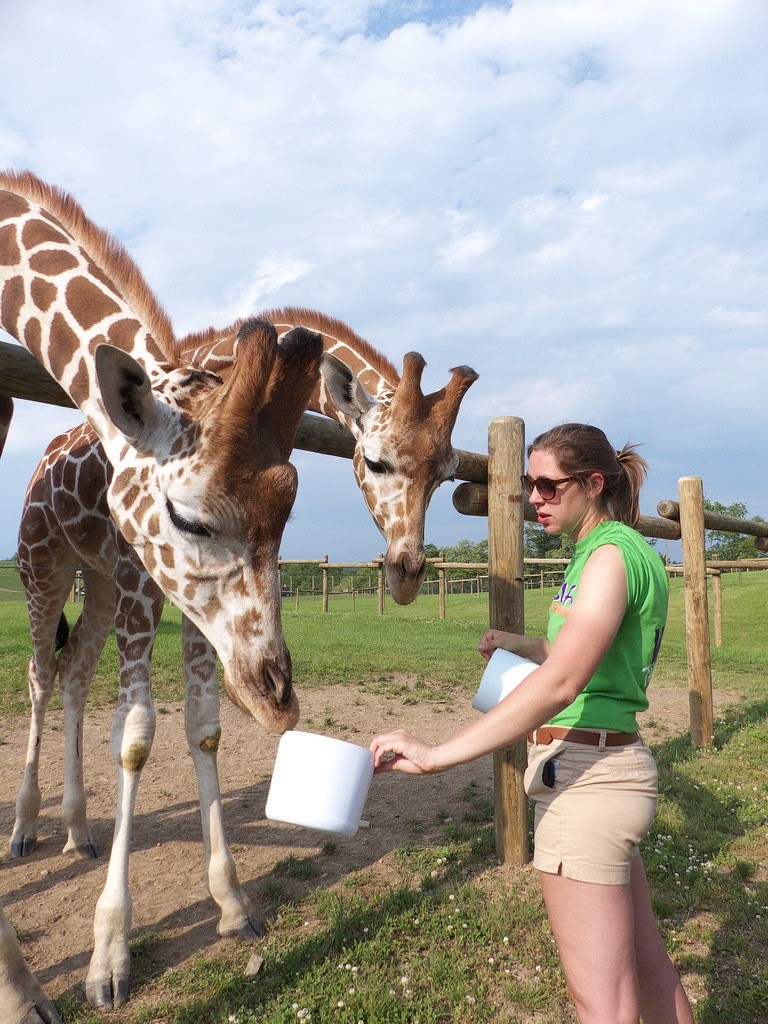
[0,171,483,1024]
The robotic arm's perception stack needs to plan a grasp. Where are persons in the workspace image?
[369,421,695,1024]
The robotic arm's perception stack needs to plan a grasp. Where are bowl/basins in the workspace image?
[471,646,540,714]
[266,728,377,836]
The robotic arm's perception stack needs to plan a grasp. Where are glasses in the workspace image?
[520,475,574,500]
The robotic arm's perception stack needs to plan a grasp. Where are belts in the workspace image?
[532,728,641,749]
